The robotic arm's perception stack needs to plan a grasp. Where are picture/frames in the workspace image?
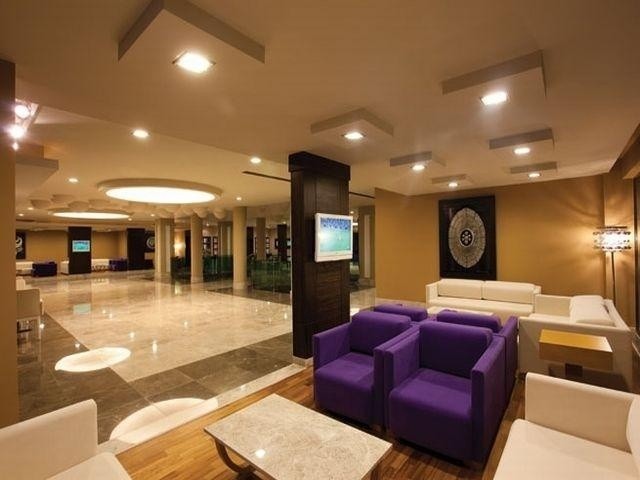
[72,240,91,253]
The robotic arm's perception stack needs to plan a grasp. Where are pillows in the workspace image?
[438,279,482,299]
[482,281,535,304]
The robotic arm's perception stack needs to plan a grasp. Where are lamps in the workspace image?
[118,0,265,105]
[593,226,631,306]
[97,179,223,205]
[310,51,558,192]
[49,208,131,220]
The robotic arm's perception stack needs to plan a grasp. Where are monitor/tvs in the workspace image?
[73,241,89,252]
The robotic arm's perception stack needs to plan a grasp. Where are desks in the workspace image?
[539,329,612,384]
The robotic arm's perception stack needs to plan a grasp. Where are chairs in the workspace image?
[16,288,41,341]
[0,399,132,480]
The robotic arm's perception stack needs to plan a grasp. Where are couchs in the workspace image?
[517,295,632,389]
[426,281,542,329]
[312,309,418,431]
[358,303,428,324]
[426,308,519,406]
[383,322,506,472]
[491,372,639,478]
